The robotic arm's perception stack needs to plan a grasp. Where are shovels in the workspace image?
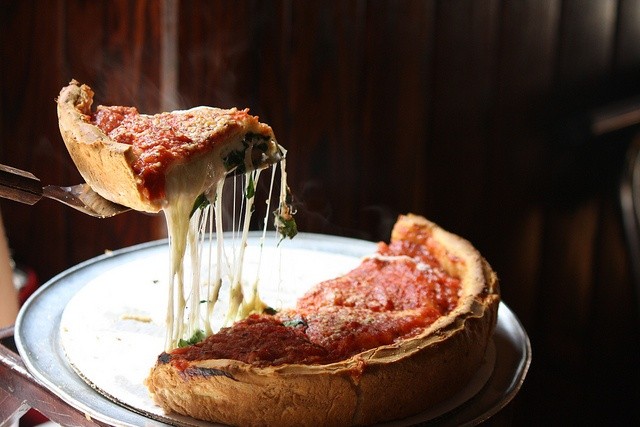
[0,142,283,218]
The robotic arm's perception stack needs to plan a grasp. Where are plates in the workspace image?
[13,229,533,427]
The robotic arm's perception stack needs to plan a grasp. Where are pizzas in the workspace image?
[56,77,299,319]
[145,211,503,427]
